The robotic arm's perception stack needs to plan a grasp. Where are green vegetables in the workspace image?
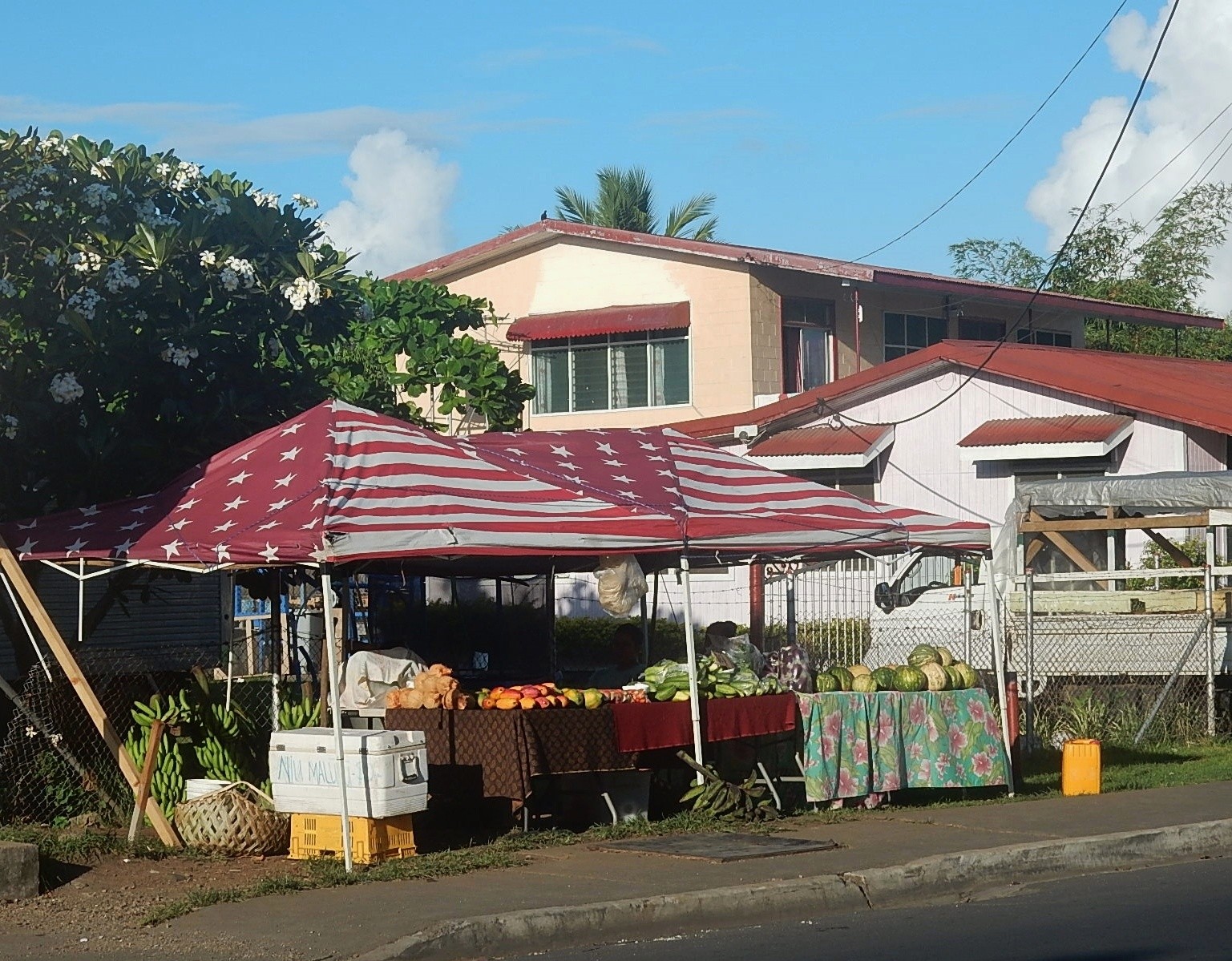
[724,641,768,676]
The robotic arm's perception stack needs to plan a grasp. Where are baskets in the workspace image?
[286,813,418,865]
[174,780,291,855]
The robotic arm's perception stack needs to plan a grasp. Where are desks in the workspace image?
[383,688,1010,834]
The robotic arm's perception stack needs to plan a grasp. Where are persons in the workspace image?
[587,627,647,691]
[702,621,738,654]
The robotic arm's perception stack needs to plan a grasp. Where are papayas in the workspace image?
[475,682,570,709]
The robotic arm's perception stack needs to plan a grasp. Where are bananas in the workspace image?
[125,689,322,826]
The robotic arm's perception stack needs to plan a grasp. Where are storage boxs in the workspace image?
[268,709,429,865]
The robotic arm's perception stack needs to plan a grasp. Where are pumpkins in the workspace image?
[813,644,977,692]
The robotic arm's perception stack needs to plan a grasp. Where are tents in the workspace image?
[0,398,1016,872]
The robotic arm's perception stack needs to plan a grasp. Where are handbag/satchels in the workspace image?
[703,621,814,694]
[593,553,649,618]
